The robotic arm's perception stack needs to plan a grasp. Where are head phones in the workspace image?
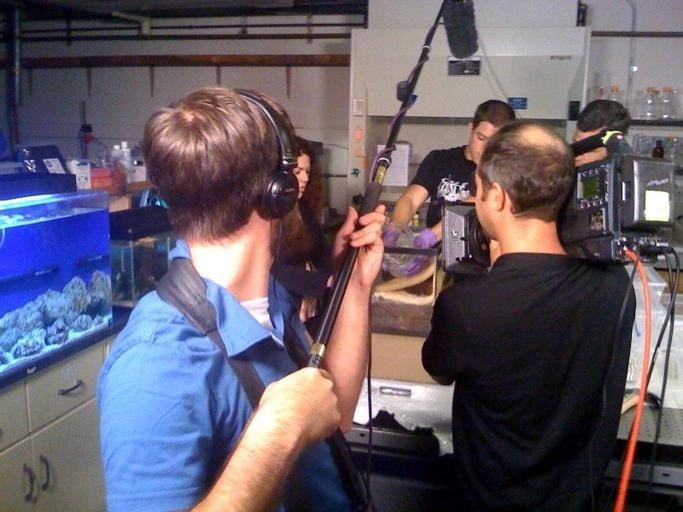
[236,90,299,223]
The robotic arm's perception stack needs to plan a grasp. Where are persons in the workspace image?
[380,100,516,276]
[422,118,637,512]
[95,86,388,512]
[572,99,632,166]
[267,136,335,339]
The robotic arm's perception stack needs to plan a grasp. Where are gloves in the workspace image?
[405,230,436,277]
[377,223,400,272]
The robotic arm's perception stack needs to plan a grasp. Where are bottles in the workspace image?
[597,84,676,120]
[111,141,132,184]
[634,134,681,173]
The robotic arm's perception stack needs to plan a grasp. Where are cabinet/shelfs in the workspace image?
[606,461,683,512]
[343,376,455,512]
[0,341,106,512]
